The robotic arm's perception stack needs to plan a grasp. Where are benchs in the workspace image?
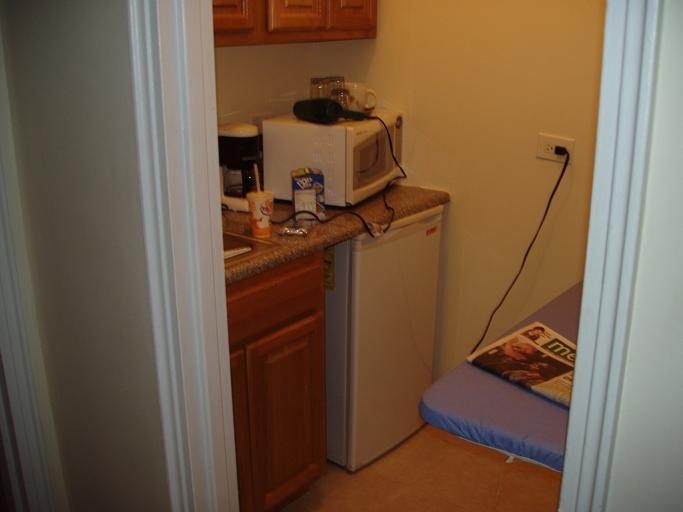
[417,280,584,474]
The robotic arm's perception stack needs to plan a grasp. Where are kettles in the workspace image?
[218,123,260,215]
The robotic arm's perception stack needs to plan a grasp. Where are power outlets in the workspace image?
[536,133,576,165]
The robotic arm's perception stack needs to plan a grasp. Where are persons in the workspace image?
[522,325,546,342]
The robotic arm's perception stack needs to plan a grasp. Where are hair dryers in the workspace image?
[292,99,367,125]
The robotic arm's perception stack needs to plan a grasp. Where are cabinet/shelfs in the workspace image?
[260,0,378,44]
[213,0,261,47]
[226,248,330,512]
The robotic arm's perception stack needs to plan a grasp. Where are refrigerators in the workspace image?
[324,205,445,473]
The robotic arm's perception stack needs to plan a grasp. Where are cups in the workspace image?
[345,78,378,112]
[245,190,276,239]
[308,76,350,109]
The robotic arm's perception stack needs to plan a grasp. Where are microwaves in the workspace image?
[262,108,405,210]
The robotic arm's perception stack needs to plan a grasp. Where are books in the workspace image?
[221,232,252,260]
